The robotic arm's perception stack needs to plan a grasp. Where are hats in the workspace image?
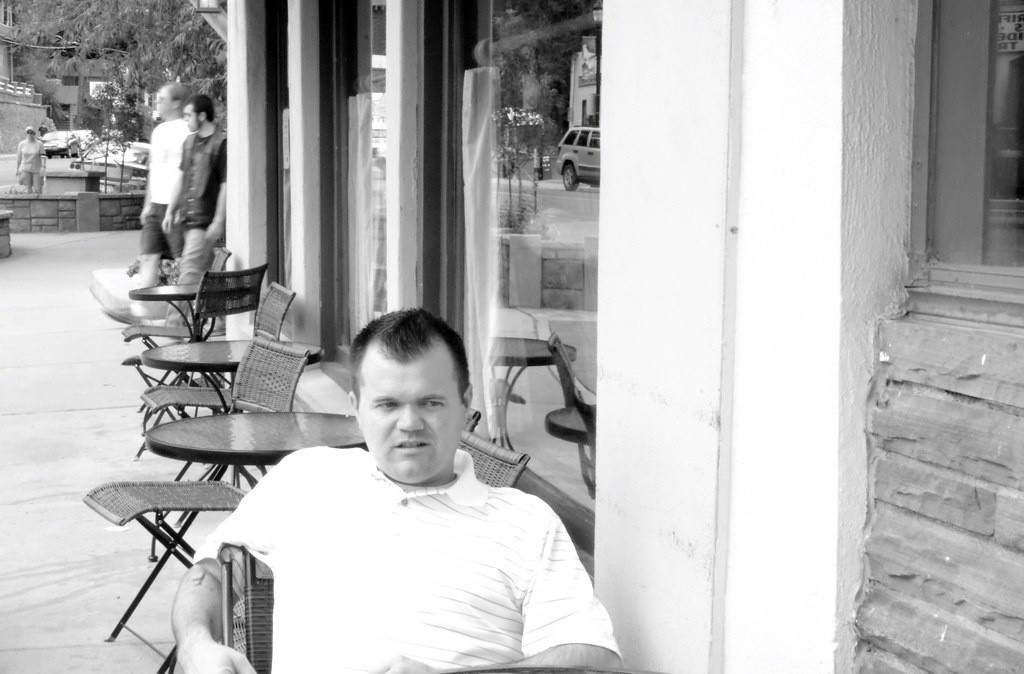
[25,126,35,134]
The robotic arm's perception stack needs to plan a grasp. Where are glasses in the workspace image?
[27,131,33,134]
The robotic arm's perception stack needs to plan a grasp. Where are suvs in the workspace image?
[555,126,600,192]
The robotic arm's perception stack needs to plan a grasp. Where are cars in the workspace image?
[72,129,101,152]
[40,130,82,158]
[81,139,152,193]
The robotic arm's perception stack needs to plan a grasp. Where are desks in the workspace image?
[545,401,595,500]
[481,336,580,451]
[129,282,226,343]
[100,411,369,649]
[135,339,253,463]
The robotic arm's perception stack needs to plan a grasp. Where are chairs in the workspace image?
[82,237,534,674]
[545,335,595,497]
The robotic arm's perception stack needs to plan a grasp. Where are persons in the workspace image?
[170,306,626,674]
[39,122,48,136]
[161,94,227,340]
[129,81,197,291]
[16,126,46,193]
